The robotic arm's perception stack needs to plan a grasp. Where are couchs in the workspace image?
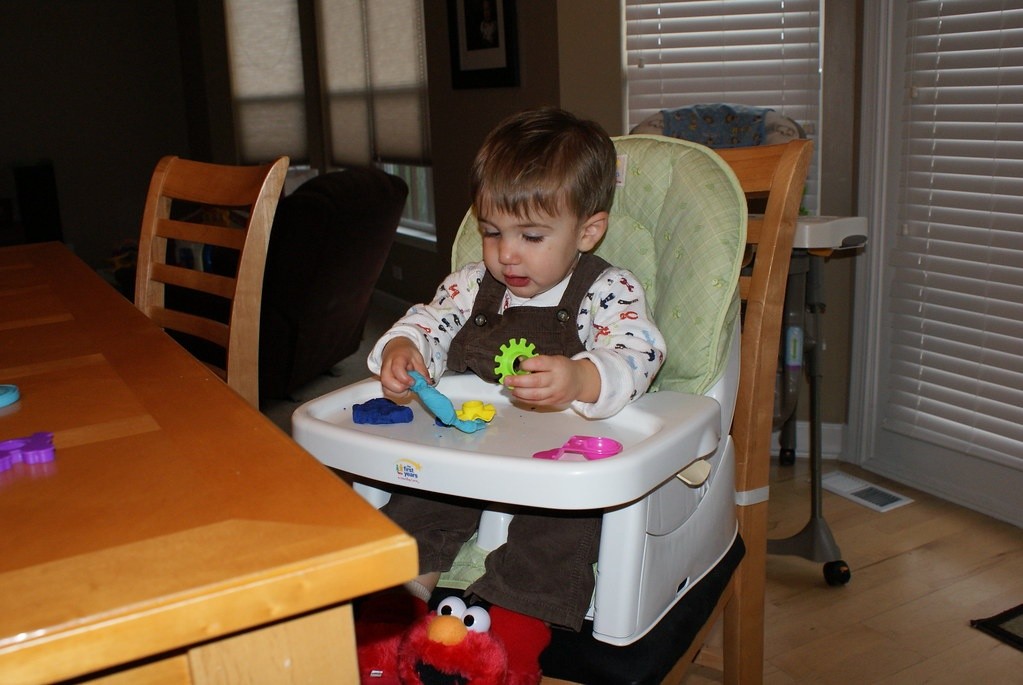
[258,168,409,402]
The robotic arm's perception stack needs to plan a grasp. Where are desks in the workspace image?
[289,373,722,509]
[0,240,419,685]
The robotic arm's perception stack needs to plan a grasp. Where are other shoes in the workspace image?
[355,584,429,685]
[398,593,551,685]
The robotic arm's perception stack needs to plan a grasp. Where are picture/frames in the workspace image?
[446,0,520,89]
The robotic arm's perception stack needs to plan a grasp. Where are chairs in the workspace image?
[629,104,868,588]
[135,155,289,410]
[291,138,812,685]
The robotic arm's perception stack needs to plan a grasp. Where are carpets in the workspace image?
[970,604,1023,652]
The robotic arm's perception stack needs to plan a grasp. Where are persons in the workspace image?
[355,106,667,685]
